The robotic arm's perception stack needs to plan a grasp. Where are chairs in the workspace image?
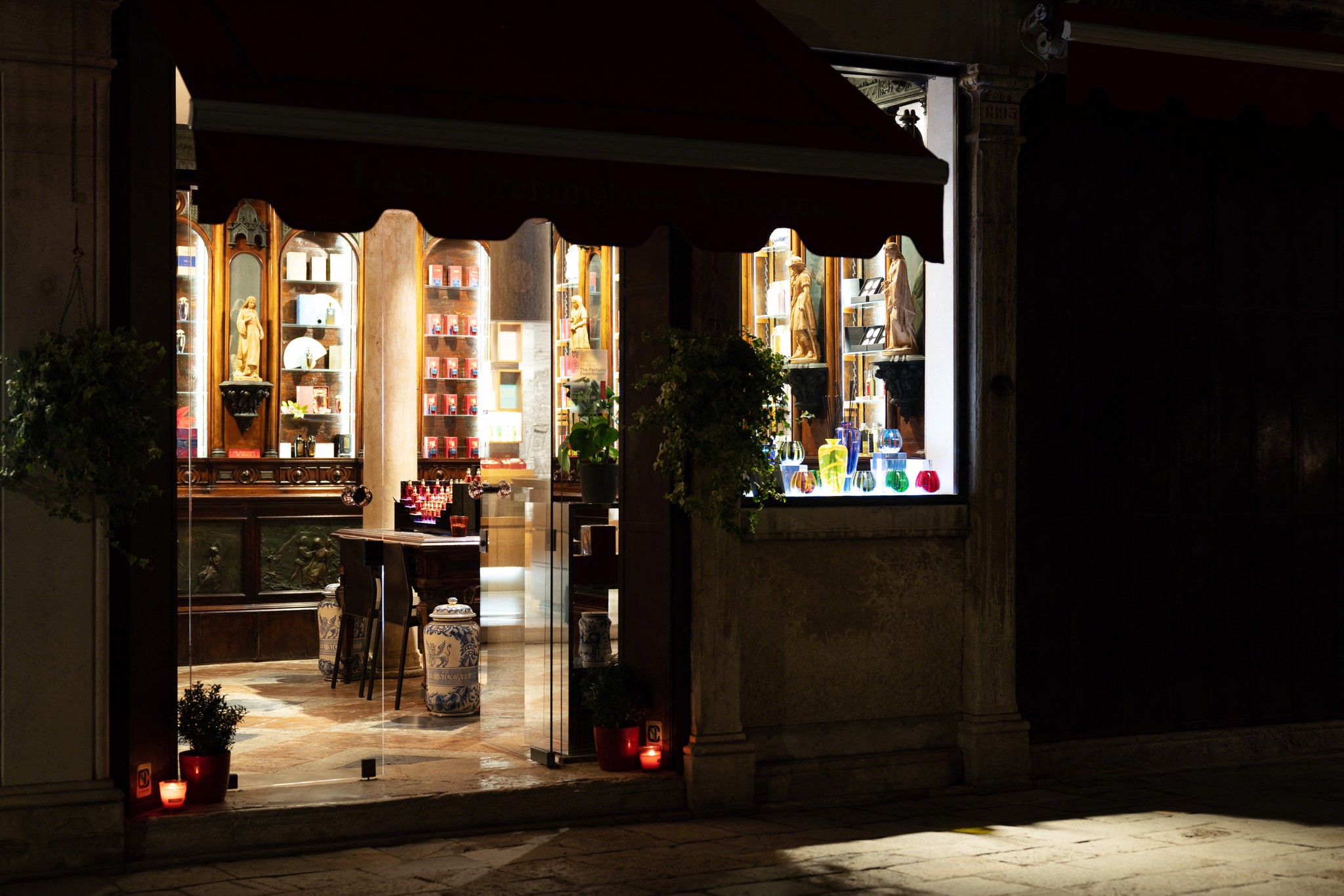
[331,534,422,710]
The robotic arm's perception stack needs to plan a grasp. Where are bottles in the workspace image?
[850,470,940,494]
[859,423,870,456]
[762,441,817,495]
[578,610,612,667]
[770,228,790,248]
[178,297,189,321]
[326,302,336,325]
[770,325,790,359]
[868,422,882,456]
[306,435,316,457]
[335,394,344,414]
[766,280,790,316]
[818,438,848,496]
[316,389,324,413]
[835,421,861,477]
[294,434,305,458]
[405,467,481,523]
[422,595,481,718]
[866,369,876,400]
[878,428,902,454]
[176,329,186,354]
[429,317,479,458]
[305,346,314,370]
[317,577,366,683]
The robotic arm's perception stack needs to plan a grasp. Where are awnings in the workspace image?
[159,1,946,265]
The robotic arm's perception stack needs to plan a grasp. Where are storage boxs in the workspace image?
[844,277,886,353]
[423,313,479,458]
[296,386,329,413]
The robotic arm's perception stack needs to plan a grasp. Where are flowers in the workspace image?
[281,401,308,419]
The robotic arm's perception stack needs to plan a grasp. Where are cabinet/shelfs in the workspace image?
[282,278,357,418]
[418,284,483,463]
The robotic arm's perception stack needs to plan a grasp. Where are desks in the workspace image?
[330,529,480,689]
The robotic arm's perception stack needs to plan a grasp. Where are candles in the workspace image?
[159,779,187,811]
[640,746,661,769]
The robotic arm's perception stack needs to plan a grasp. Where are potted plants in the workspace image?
[557,377,619,503]
[176,681,250,803]
[580,652,651,772]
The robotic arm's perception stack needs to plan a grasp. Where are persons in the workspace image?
[881,242,916,350]
[568,296,594,374]
[912,260,925,356]
[230,299,244,364]
[785,256,818,359]
[236,295,265,378]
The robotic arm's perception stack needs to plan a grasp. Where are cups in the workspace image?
[159,779,188,814]
[450,516,469,537]
[638,746,662,772]
